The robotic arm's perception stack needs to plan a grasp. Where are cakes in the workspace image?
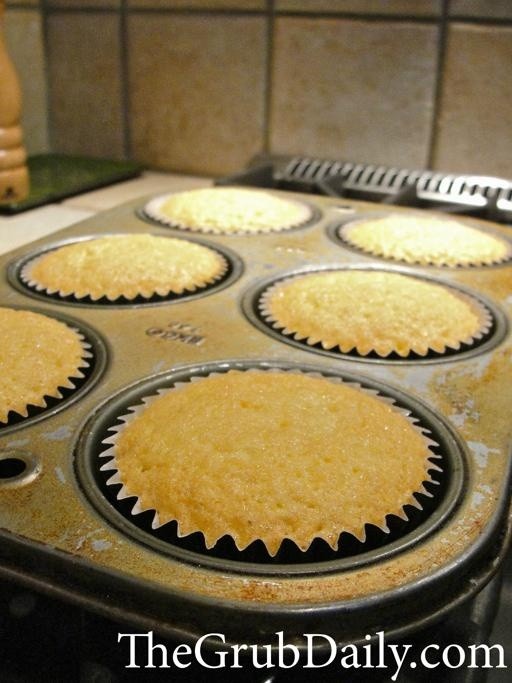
[159,187,304,235]
[343,212,507,269]
[28,232,222,303]
[266,269,485,357]
[115,369,430,556]
[0,305,85,423]
